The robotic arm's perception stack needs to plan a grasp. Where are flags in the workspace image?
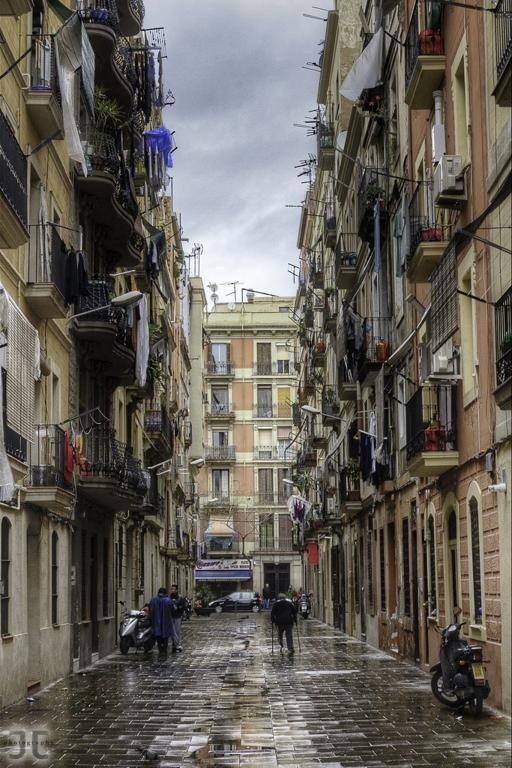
[292,495,312,525]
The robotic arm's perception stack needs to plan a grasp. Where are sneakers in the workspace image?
[158,644,182,652]
[289,648,295,652]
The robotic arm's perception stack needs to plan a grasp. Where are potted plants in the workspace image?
[90,83,127,171]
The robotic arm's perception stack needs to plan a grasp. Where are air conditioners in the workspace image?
[419,333,455,383]
[312,287,325,311]
[432,154,466,204]
[326,498,336,516]
[177,453,183,468]
[176,507,184,520]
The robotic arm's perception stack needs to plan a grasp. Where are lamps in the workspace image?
[156,458,205,476]
[300,405,347,426]
[282,477,321,495]
[65,290,143,328]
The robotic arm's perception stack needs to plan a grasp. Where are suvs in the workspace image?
[208,590,263,612]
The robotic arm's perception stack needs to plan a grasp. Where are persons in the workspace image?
[262,582,271,609]
[270,590,298,653]
[169,583,186,652]
[147,586,178,662]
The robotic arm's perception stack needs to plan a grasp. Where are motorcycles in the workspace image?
[182,596,194,620]
[194,596,206,617]
[118,600,155,653]
[297,591,312,619]
[426,606,493,719]
